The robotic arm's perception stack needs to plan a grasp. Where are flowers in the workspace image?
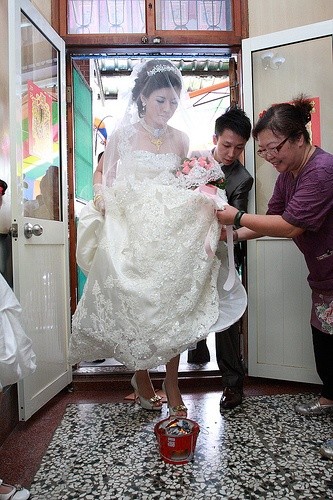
[171,149,224,189]
[213,178,230,189]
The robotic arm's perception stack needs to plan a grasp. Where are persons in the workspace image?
[92,61,247,418]
[216,94,333,459]
[186,108,255,410]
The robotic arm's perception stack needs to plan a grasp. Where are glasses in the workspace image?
[257,133,294,158]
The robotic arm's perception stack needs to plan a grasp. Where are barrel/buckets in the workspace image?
[154,417,200,464]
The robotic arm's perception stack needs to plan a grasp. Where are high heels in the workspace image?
[131,371,163,410]
[162,379,188,418]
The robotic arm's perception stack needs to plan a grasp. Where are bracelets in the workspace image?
[234,210,247,229]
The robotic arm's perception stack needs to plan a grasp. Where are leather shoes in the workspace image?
[295,399,333,416]
[320,438,333,460]
[220,387,245,409]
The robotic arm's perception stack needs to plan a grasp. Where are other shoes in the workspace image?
[0,479,30,500]
